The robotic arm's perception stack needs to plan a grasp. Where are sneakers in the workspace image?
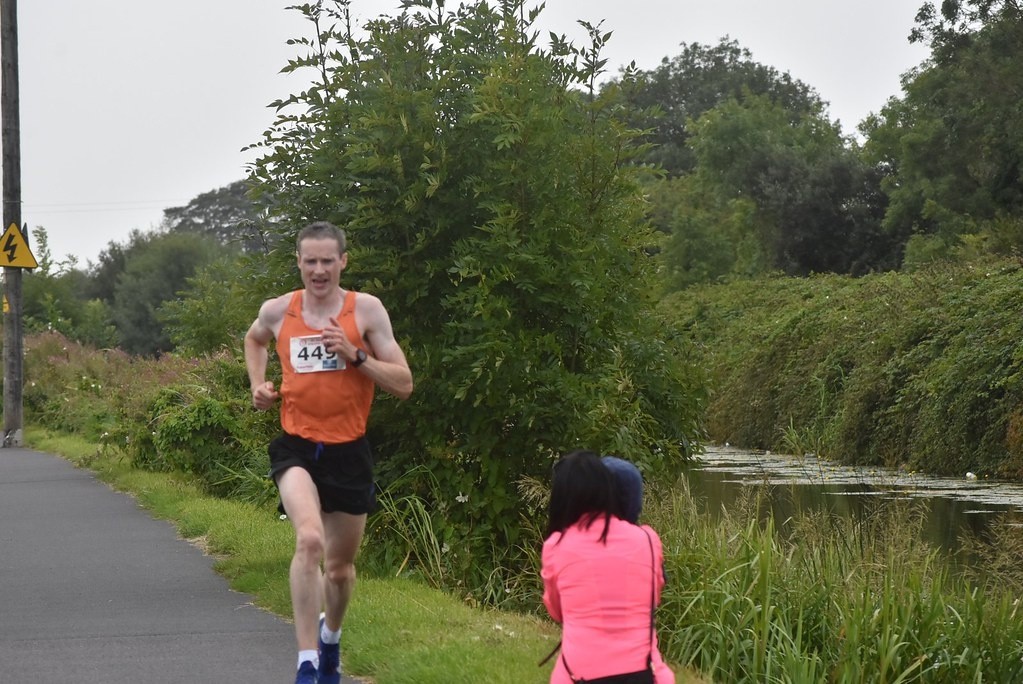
[318,611,341,684]
[294,660,320,684]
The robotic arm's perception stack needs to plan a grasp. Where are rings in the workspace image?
[327,339,330,346]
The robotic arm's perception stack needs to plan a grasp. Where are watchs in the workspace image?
[351,349,367,367]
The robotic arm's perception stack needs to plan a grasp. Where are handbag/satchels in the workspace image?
[573,669,656,684]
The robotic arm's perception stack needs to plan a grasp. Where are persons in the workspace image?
[603,456,643,525]
[245,222,414,684]
[540,452,676,684]
